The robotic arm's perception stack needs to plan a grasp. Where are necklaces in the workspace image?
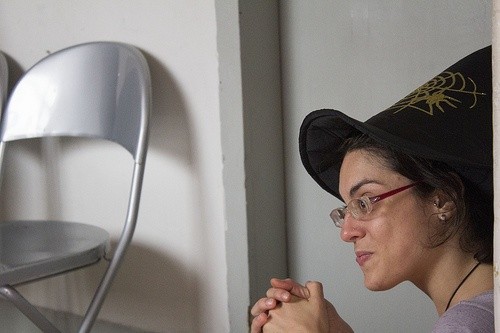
[445,260,482,312]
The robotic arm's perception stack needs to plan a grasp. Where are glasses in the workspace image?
[329,183,417,227]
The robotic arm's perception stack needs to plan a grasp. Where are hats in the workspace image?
[299,44,493,203]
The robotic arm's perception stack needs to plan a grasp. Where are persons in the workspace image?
[250,44,494,333]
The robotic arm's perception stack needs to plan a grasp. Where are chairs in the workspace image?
[0,40,153,333]
